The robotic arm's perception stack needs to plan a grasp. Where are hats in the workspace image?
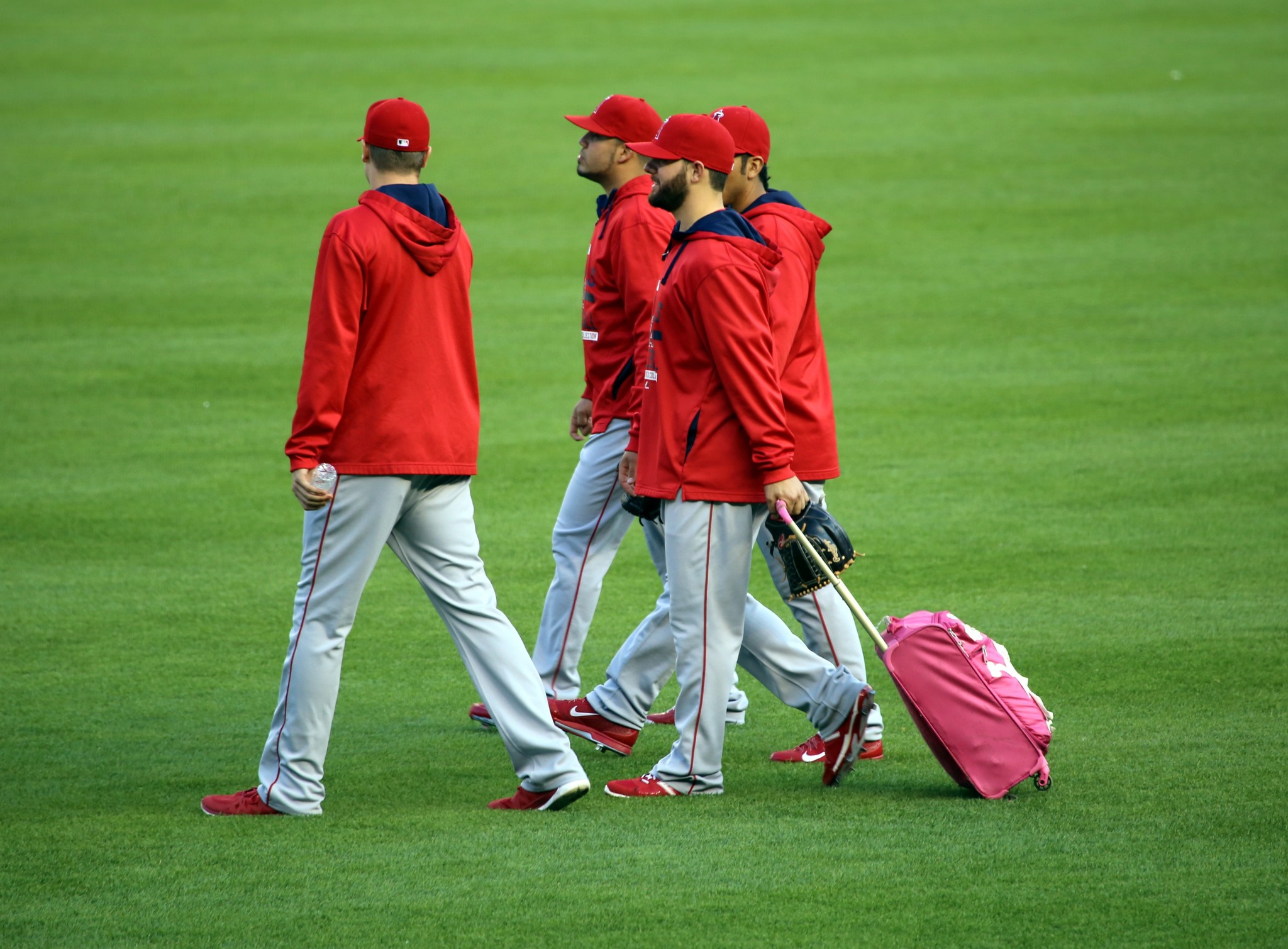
[356,97,430,153]
[562,95,664,143]
[627,112,734,175]
[708,105,768,165]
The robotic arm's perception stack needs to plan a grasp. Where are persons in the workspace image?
[464,93,885,799]
[199,96,592,821]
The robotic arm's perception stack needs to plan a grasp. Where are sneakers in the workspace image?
[822,683,875,786]
[545,698,640,756]
[486,778,589,813]
[602,775,679,798]
[467,701,496,725]
[200,786,281,818]
[647,702,744,726]
[769,731,883,762]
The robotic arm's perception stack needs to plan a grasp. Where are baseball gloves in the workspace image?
[621,490,663,525]
[765,498,855,599]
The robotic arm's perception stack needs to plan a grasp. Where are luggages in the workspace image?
[775,500,1055,798]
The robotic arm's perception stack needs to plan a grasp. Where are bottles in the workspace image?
[309,463,338,493]
